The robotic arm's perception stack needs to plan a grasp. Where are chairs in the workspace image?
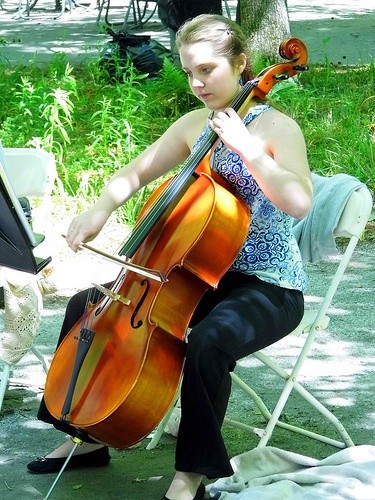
[0,145,57,419]
[144,170,372,463]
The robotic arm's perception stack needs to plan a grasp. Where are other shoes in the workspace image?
[161,481,206,500]
[27,444,110,474]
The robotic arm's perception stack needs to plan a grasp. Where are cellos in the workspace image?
[41,34,307,500]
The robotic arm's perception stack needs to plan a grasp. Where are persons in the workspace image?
[26,12,314,500]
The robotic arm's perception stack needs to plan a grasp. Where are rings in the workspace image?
[215,128,223,137]
